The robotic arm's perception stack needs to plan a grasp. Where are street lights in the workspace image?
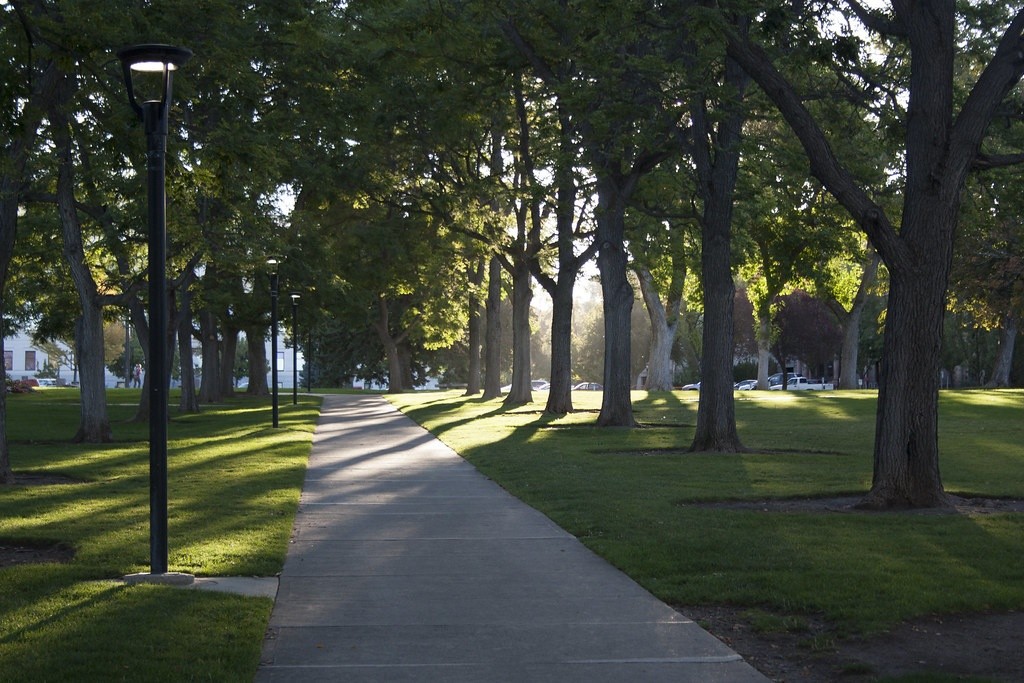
[288,290,302,405]
[265,254,286,430]
[116,42,198,573]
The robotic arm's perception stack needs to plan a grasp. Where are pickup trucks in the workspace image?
[769,376,835,391]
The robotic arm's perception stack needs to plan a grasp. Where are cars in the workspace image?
[682,381,701,390]
[571,382,603,391]
[531,379,550,390]
[732,372,828,392]
